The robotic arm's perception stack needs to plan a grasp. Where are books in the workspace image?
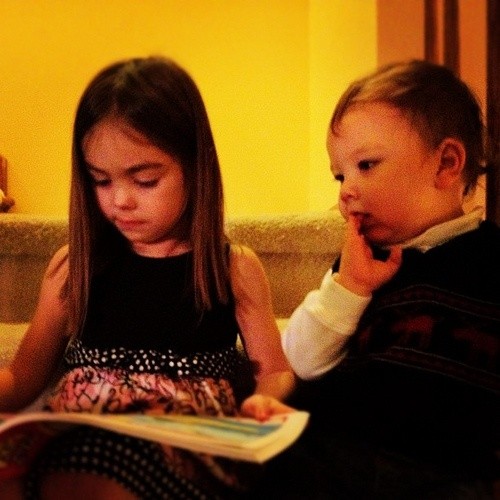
[0,411,310,463]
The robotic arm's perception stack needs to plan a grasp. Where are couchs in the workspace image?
[0,213,349,360]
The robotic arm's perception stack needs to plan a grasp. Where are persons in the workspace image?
[281,60,499,500]
[0,56,300,499]
